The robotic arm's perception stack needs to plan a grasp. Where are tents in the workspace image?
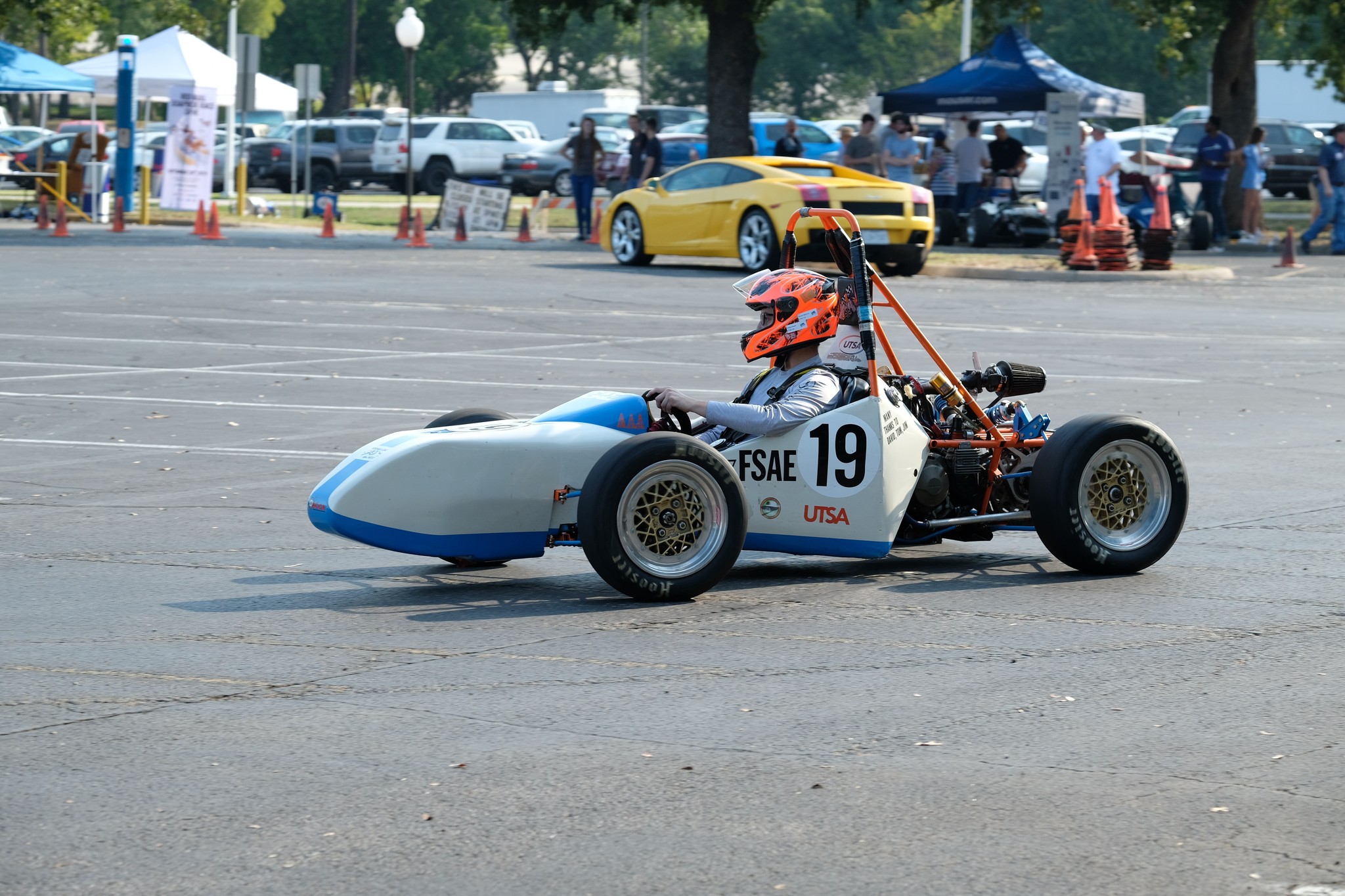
[877,25,1147,178]
[62,23,300,196]
[0,43,98,223]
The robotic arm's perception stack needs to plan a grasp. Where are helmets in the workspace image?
[740,268,840,364]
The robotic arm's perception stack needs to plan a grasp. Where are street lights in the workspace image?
[396,6,425,225]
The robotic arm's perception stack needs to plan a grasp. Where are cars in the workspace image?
[1163,105,1215,126]
[1306,122,1339,143]
[566,105,709,145]
[599,133,708,190]
[1,106,273,187]
[658,116,845,167]
[500,137,580,198]
[813,114,1203,197]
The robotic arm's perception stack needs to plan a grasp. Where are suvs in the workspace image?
[345,107,409,121]
[235,116,377,193]
[369,116,555,197]
[1165,116,1328,199]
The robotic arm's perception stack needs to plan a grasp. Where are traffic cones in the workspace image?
[106,197,130,233]
[49,198,76,238]
[1051,179,1180,273]
[33,195,54,229]
[514,207,536,243]
[449,206,471,242]
[391,204,432,248]
[188,201,228,239]
[586,208,605,244]
[1274,228,1307,268]
[316,203,338,238]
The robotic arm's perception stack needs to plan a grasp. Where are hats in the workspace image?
[1091,120,1113,133]
[637,115,661,134]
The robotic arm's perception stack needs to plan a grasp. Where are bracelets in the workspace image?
[1212,161,1217,166]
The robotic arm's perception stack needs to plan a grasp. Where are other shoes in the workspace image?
[1332,250,1345,255]
[1300,235,1311,254]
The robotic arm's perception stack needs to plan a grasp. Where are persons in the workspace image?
[834,110,1027,247]
[1193,116,1236,244]
[1078,120,1121,225]
[621,114,663,191]
[1299,123,1345,256]
[773,118,803,158]
[645,267,844,451]
[559,116,607,241]
[1236,126,1267,240]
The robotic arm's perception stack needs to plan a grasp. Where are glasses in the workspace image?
[760,312,773,325]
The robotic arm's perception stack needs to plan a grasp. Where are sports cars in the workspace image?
[601,154,937,272]
